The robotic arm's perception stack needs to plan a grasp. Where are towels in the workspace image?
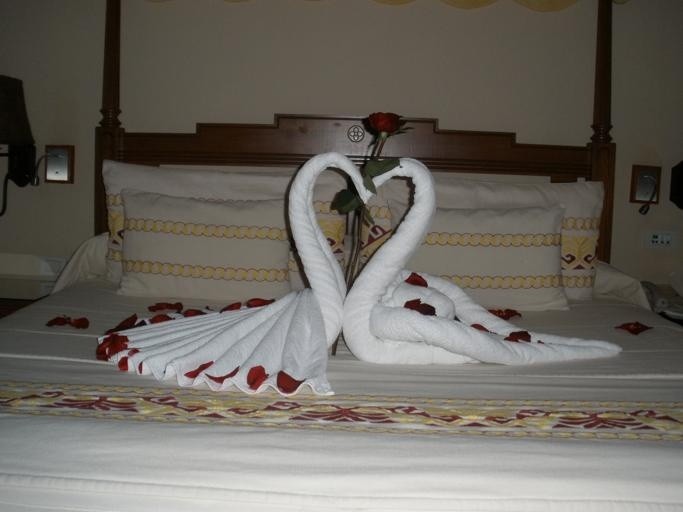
[97,288,335,396]
[342,156,481,365]
[287,151,367,350]
[370,269,623,367]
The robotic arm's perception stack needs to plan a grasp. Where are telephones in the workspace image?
[640,281,683,326]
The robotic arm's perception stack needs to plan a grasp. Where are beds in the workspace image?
[0,113,683,512]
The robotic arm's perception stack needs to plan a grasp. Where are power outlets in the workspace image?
[647,229,672,248]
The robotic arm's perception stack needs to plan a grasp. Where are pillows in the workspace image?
[102,159,347,292]
[115,188,291,302]
[387,197,570,311]
[358,181,605,301]
